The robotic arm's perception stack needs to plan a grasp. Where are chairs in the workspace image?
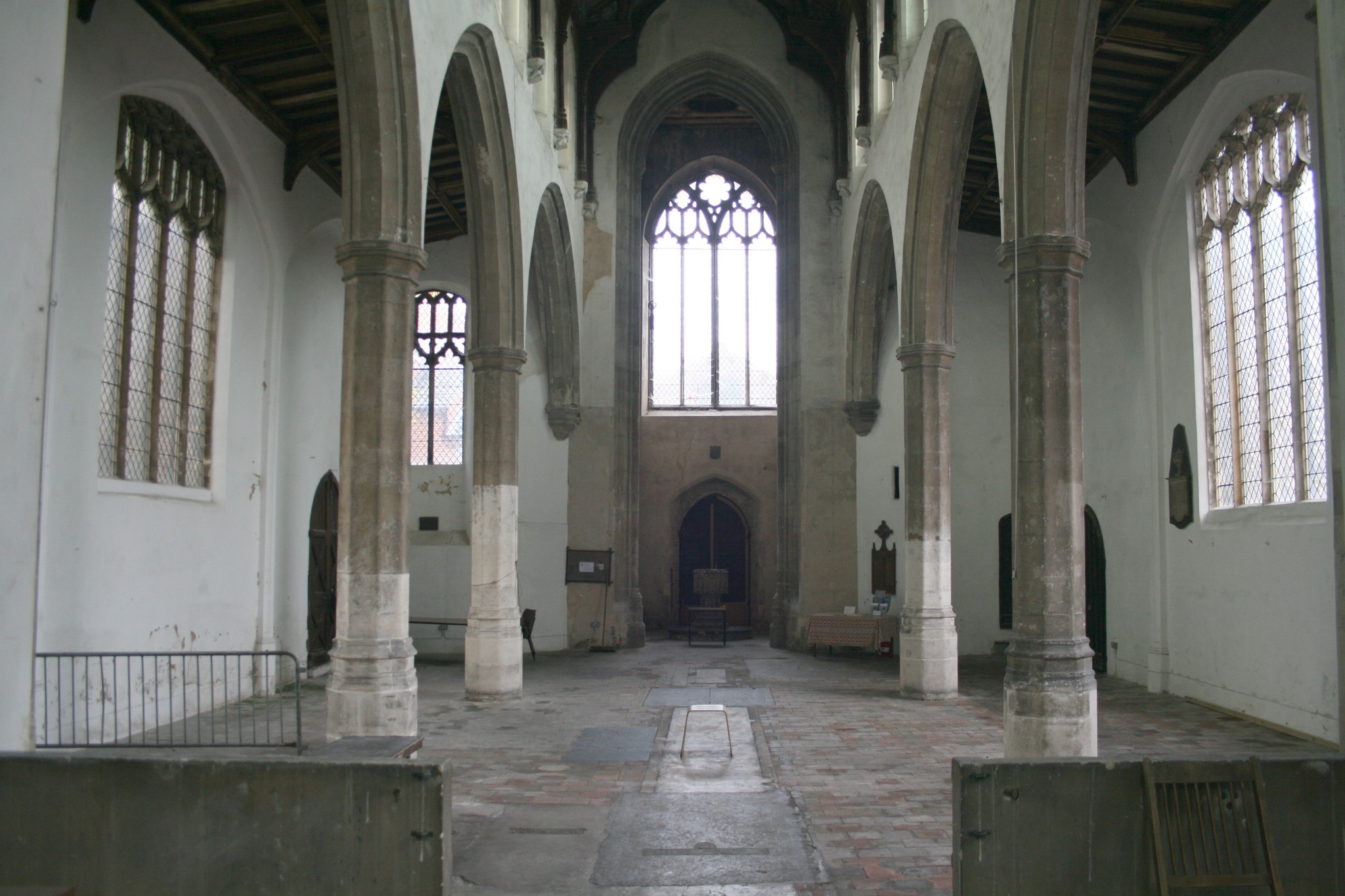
[687,569,730,647]
[520,608,536,661]
[1142,754,1285,896]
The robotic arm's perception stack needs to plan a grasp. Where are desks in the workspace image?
[304,735,426,758]
[806,613,898,659]
[409,617,467,625]
